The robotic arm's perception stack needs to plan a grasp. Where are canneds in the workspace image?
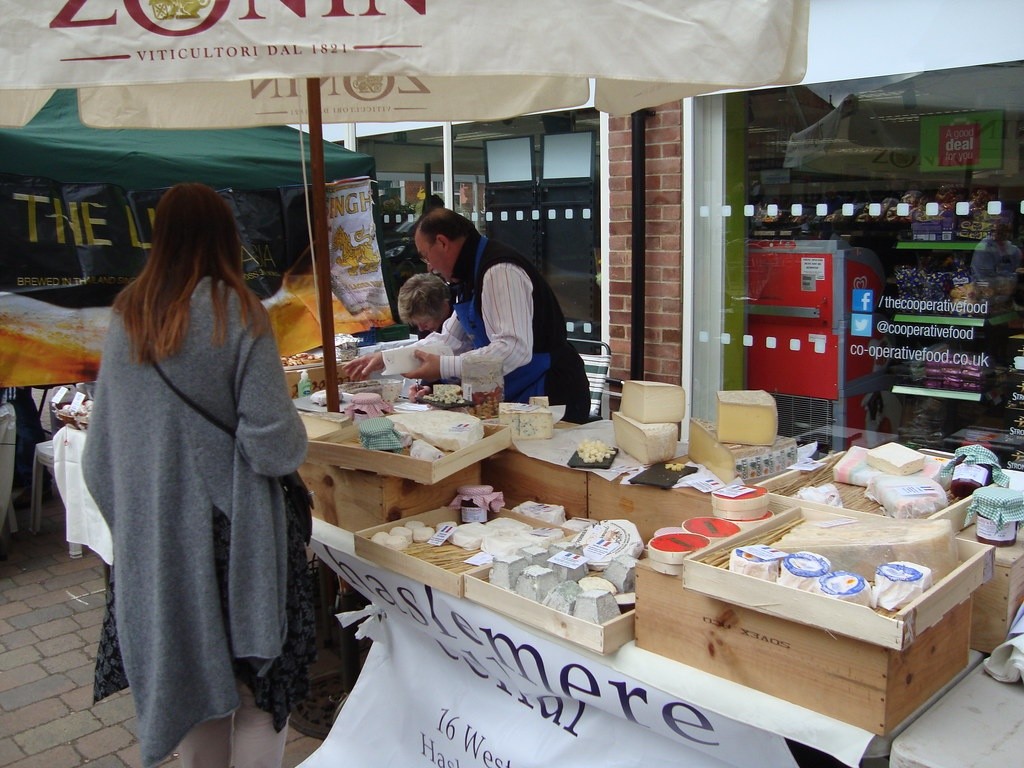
[344,392,410,454]
[940,445,1024,547]
[457,485,505,523]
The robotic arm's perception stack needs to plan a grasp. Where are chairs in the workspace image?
[29,441,83,559]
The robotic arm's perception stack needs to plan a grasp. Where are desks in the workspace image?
[53,424,1024,768]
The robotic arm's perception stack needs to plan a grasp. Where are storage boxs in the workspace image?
[480,450,588,521]
[955,522,1024,656]
[634,557,972,735]
[296,463,482,533]
[588,472,714,545]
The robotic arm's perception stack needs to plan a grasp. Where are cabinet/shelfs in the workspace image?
[890,240,1020,402]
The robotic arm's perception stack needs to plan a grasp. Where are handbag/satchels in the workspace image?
[279,471,317,546]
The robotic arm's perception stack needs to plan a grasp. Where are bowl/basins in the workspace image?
[342,378,402,406]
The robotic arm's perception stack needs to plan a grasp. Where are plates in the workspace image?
[627,461,699,491]
[415,395,477,410]
[567,447,620,468]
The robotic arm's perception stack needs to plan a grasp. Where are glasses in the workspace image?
[419,242,432,265]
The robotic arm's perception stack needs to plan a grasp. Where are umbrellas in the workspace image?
[784,70,1024,188]
[1,2,1024,411]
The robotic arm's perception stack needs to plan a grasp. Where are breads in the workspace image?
[369,501,641,625]
[382,382,926,477]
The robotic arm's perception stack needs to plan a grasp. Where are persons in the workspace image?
[398,195,445,280]
[341,207,591,424]
[82,185,309,768]
[398,273,463,404]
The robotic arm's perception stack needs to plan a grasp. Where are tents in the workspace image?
[0,91,379,310]
